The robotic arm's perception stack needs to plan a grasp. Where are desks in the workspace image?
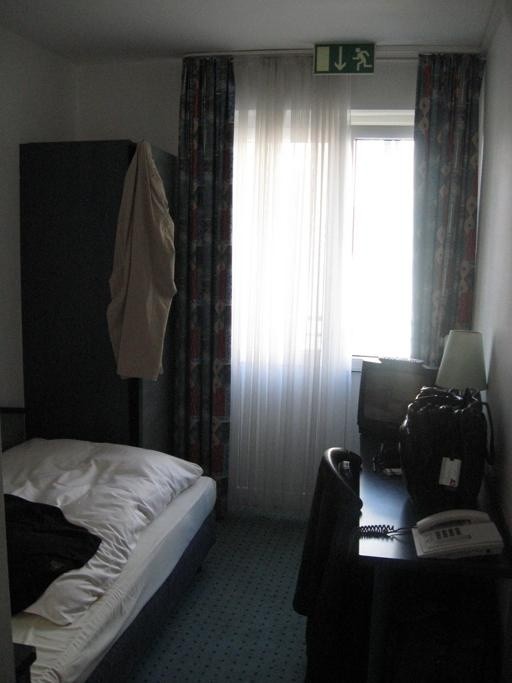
[359,433,512,683]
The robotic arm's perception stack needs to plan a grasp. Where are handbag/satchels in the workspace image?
[398,384,495,514]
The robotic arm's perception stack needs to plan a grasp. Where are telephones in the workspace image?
[412,510,504,559]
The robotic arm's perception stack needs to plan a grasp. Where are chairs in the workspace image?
[293,448,371,682]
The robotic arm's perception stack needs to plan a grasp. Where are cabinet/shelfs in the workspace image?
[20,140,177,452]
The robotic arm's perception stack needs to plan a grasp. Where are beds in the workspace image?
[2,437,217,683]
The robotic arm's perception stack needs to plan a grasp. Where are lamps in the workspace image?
[435,330,488,396]
